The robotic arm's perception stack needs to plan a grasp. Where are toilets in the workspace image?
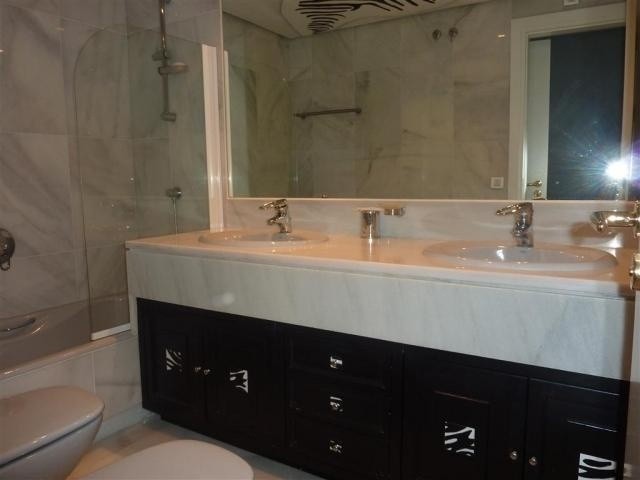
[0,385,255,480]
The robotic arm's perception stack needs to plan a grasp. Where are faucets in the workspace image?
[258,199,292,234]
[496,200,533,247]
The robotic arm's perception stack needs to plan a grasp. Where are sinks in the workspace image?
[420,240,619,272]
[196,230,331,247]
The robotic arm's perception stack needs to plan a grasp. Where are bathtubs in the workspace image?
[1,292,132,381]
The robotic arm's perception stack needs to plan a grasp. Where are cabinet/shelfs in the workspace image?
[136,296,287,462]
[286,323,404,480]
[404,343,631,480]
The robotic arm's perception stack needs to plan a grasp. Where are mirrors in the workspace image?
[215,0,637,202]
